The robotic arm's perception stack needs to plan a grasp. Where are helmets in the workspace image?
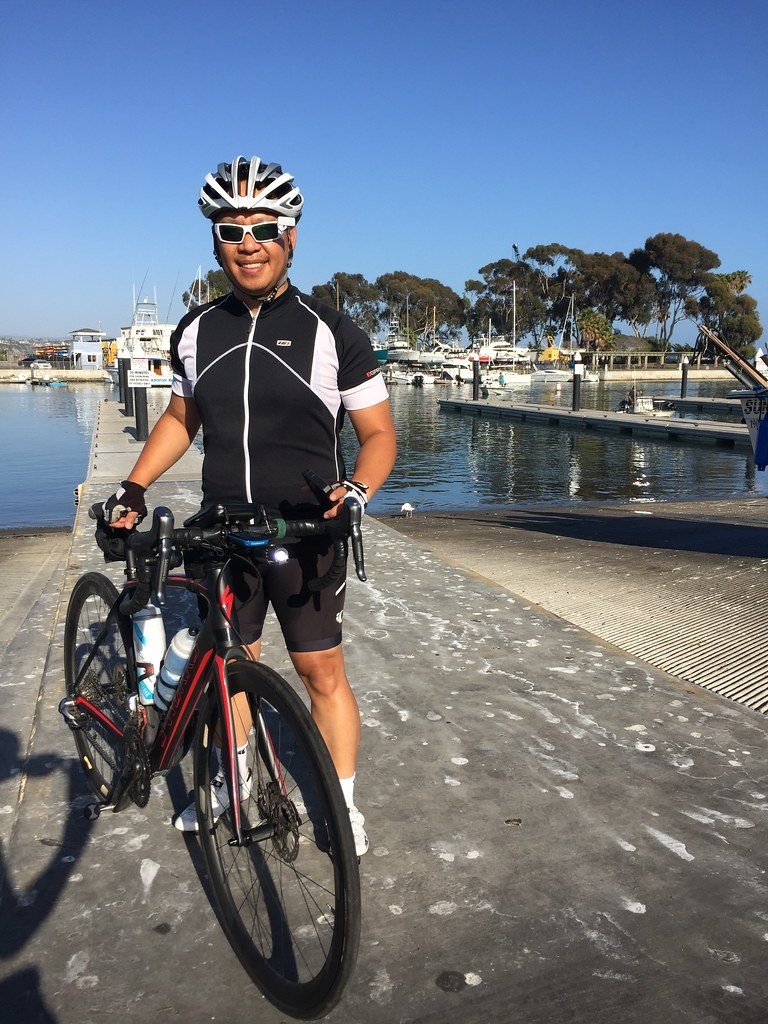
[197,155,305,227]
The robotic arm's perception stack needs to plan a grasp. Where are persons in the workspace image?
[102,154,397,856]
[628,386,637,404]
[498,372,504,385]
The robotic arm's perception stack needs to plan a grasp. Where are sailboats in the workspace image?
[370,279,601,387]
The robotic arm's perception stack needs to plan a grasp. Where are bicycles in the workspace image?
[58,497,370,1023]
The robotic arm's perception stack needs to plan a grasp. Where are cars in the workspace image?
[30,359,52,369]
[18,358,36,367]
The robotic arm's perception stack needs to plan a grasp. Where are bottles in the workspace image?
[154,626,200,712]
[132,599,167,705]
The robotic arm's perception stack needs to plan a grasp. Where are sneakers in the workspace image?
[174,768,252,832]
[325,805,370,856]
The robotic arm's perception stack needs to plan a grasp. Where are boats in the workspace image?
[104,271,183,387]
[606,364,677,418]
[24,377,69,388]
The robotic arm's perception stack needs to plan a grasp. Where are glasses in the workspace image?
[212,220,287,244]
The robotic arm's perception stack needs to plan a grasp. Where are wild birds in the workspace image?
[400,503,419,518]
